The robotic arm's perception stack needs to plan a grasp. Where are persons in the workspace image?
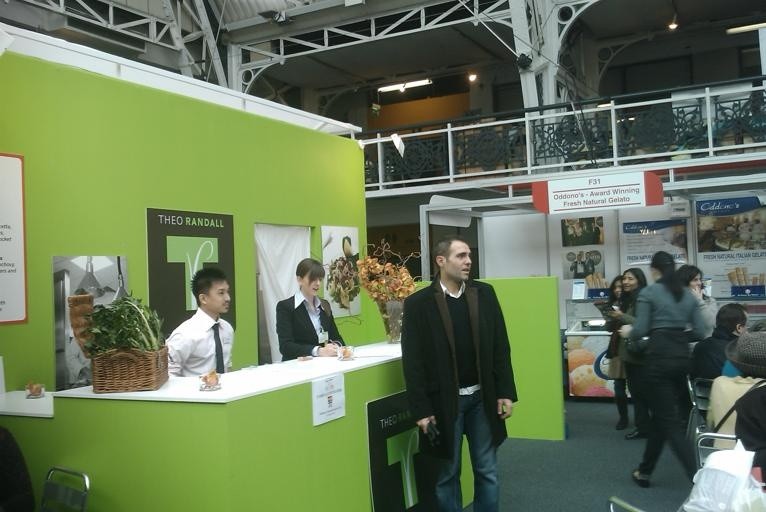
[570,252,596,279]
[276,259,351,363]
[726,213,763,243]
[165,268,235,378]
[509,123,520,147]
[402,239,518,511]
[563,221,601,247]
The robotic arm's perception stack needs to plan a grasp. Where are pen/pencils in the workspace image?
[330,339,335,345]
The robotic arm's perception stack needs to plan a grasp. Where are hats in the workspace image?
[725,329,765,376]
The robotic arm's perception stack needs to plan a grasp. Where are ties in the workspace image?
[212,323,227,374]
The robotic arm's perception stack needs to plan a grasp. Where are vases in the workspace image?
[384,300,403,344]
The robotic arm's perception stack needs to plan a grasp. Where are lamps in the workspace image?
[377,77,432,94]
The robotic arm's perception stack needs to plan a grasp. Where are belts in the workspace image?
[456,383,482,397]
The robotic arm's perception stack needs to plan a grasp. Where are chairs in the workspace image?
[695,433,737,469]
[38,467,90,512]
[608,496,644,512]
[685,374,713,440]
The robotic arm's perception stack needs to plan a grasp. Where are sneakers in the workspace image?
[616,415,651,487]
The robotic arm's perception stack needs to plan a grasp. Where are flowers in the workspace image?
[355,239,426,304]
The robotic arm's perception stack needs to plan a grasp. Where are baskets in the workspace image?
[90,344,171,393]
